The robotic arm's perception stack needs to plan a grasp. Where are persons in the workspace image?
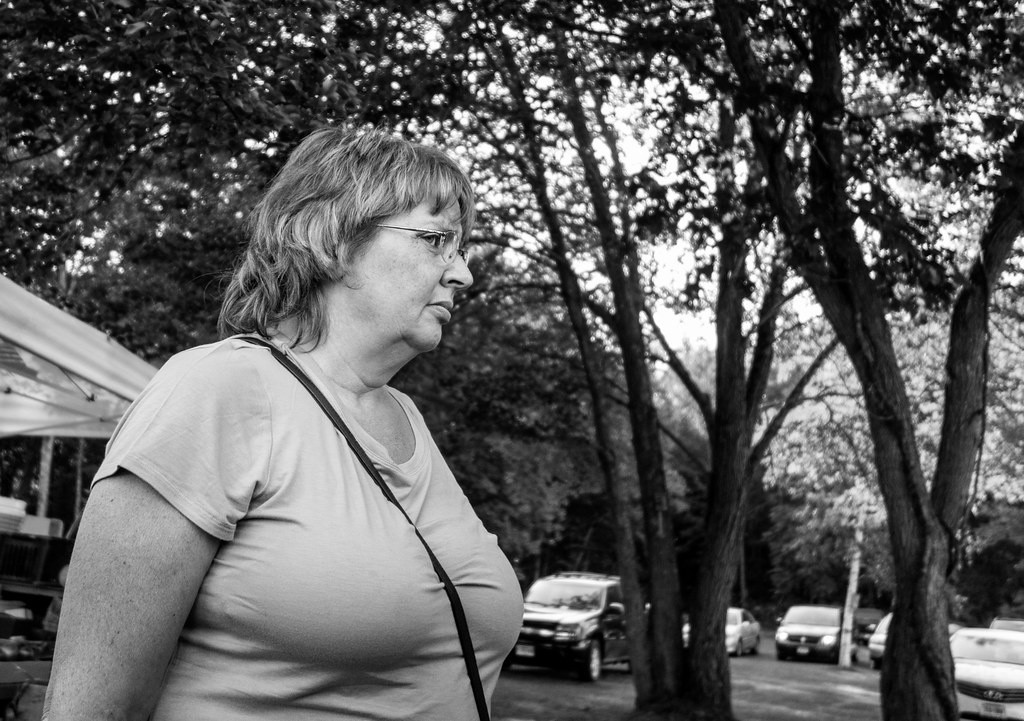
[42,128,523,721]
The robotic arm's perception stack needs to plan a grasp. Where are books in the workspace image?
[0,496,27,532]
[17,515,63,538]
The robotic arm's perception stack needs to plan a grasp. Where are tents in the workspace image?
[0,270,158,516]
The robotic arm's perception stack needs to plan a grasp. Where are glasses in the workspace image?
[373,225,473,267]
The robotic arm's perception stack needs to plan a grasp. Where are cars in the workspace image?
[868,613,892,667]
[681,608,763,656]
[851,607,885,641]
[773,604,858,662]
[948,628,1024,720]
[988,616,1024,631]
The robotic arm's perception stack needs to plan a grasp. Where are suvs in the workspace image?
[502,572,633,680]
